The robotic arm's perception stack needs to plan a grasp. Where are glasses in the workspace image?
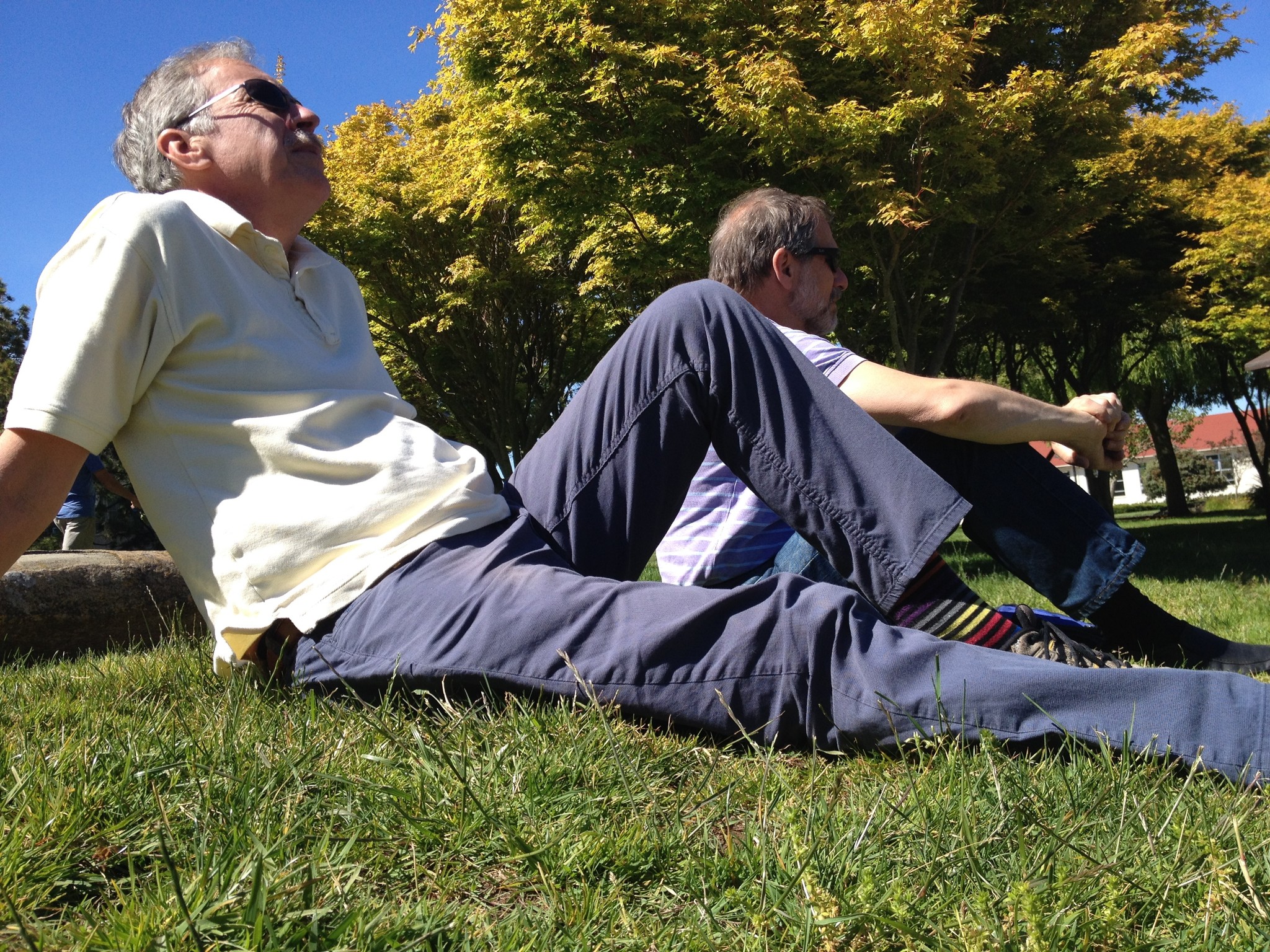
[179,76,302,115]
[795,244,843,272]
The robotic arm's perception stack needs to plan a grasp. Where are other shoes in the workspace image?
[991,603,1143,667]
[1126,619,1270,672]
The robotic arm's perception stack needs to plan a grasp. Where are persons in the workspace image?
[0,36,1270,784]
[654,188,1270,673]
[53,454,141,550]
